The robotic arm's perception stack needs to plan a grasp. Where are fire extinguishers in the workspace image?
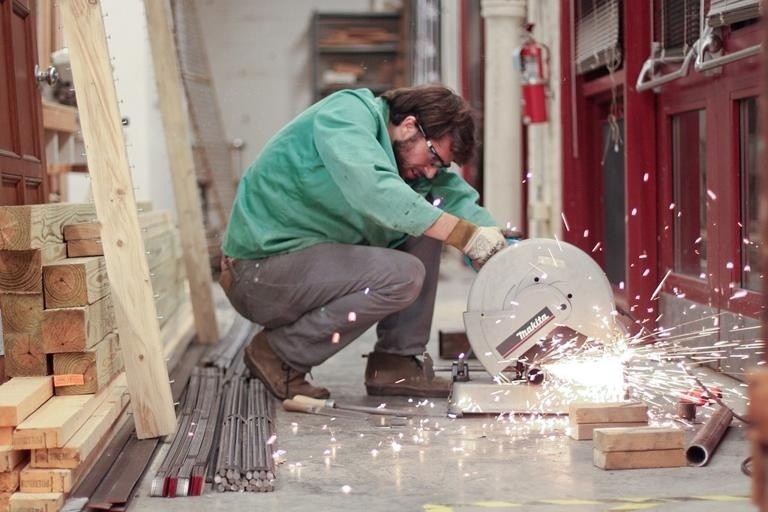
[513,24,551,123]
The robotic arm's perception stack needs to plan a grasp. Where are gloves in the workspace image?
[461,227,524,268]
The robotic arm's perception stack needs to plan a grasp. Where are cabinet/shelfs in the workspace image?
[309,9,410,101]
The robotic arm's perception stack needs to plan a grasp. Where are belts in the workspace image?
[218,269,233,296]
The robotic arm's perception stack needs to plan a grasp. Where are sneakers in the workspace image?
[243,330,330,402]
[363,354,452,398]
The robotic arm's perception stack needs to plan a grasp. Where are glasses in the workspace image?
[416,122,450,168]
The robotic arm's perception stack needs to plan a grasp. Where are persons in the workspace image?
[219,84,523,401]
